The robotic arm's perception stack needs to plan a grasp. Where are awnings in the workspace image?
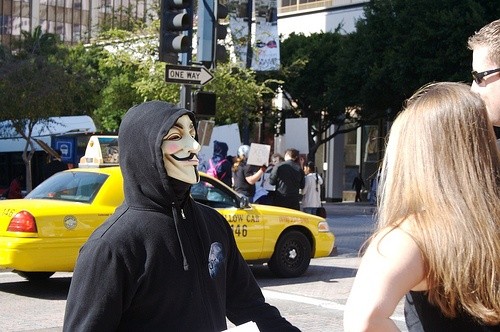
[0,114,97,151]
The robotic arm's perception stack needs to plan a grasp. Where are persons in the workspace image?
[63,99,301,332]
[468,20,500,151]
[207,140,324,215]
[8,150,67,198]
[351,173,366,203]
[340,82,500,332]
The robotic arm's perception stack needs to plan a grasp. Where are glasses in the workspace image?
[466,68,500,84]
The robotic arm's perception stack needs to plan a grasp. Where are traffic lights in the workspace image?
[214,0,228,69]
[158,0,194,64]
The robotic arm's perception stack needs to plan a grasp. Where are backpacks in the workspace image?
[204,158,228,189]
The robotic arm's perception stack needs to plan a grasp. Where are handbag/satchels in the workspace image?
[316,208,326,219]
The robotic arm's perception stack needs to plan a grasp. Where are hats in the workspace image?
[237,145,250,159]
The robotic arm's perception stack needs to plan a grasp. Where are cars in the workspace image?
[0,133,336,281]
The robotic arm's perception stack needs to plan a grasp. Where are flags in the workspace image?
[251,0,281,71]
[230,0,250,67]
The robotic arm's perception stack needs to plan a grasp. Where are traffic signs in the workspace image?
[165,65,215,85]
